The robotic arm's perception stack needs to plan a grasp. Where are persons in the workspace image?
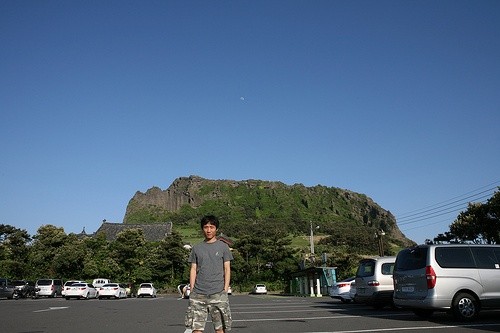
[182,285,187,299]
[185,215,234,333]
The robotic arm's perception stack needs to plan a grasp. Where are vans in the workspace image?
[92,278,111,290]
[34,278,67,298]
[392,243,500,324]
[354,256,402,311]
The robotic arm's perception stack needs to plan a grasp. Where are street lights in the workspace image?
[311,225,320,254]
[380,229,385,257]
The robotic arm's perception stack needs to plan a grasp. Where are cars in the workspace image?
[177,283,191,298]
[329,275,357,303]
[61,280,87,298]
[0,279,41,300]
[348,281,356,302]
[98,283,127,299]
[227,285,232,295]
[137,283,158,298]
[65,283,99,300]
[123,283,138,298]
[252,284,268,295]
